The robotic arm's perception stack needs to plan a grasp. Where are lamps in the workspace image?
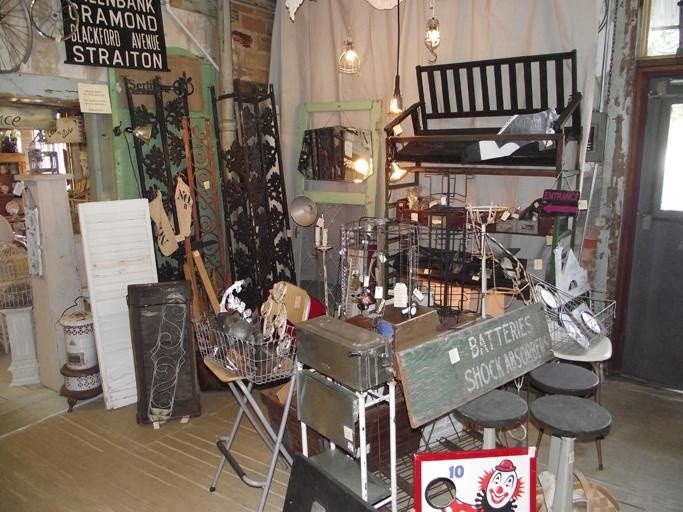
[339,0,441,114]
[123,125,154,198]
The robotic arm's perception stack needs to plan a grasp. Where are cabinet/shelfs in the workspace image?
[387,169,581,317]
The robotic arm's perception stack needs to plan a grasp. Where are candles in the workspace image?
[315,214,328,249]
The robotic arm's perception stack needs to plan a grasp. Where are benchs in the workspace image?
[386,50,583,174]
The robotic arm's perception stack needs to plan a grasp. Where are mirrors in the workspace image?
[294,100,383,314]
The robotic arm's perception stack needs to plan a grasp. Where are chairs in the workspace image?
[203,281,312,512]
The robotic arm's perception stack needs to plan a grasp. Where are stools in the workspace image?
[455,336,614,511]
